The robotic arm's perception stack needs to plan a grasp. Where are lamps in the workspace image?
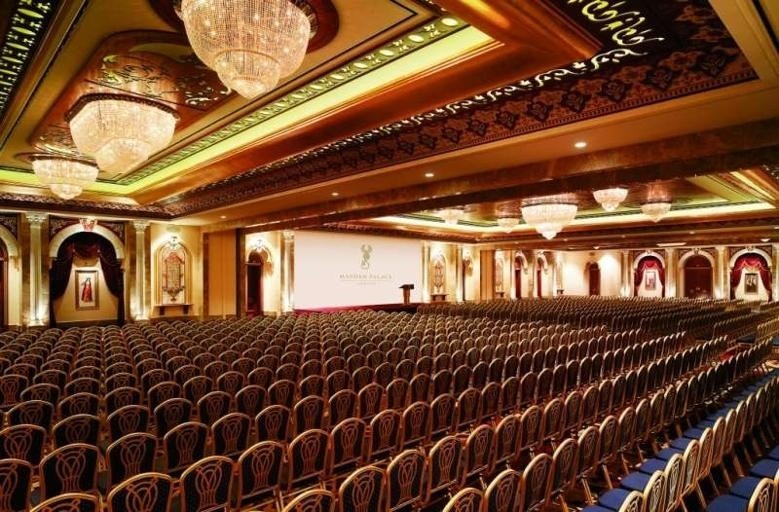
[433,180,674,240]
